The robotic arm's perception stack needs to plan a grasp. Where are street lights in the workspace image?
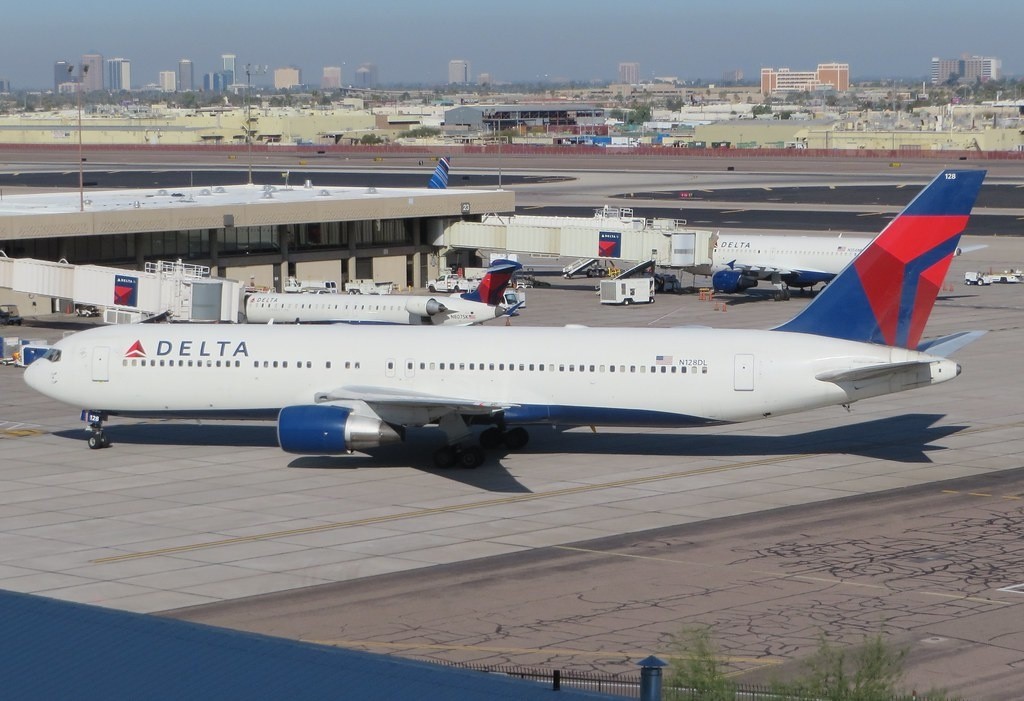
[55,59,89,211]
[245,64,268,185]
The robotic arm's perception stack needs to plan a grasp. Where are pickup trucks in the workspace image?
[428,274,482,293]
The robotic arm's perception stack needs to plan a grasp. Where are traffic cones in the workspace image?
[722,303,727,312]
[699,291,706,300]
[708,292,712,301]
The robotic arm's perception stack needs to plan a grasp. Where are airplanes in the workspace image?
[242,259,524,324]
[680,237,988,301]
[23,169,987,470]
